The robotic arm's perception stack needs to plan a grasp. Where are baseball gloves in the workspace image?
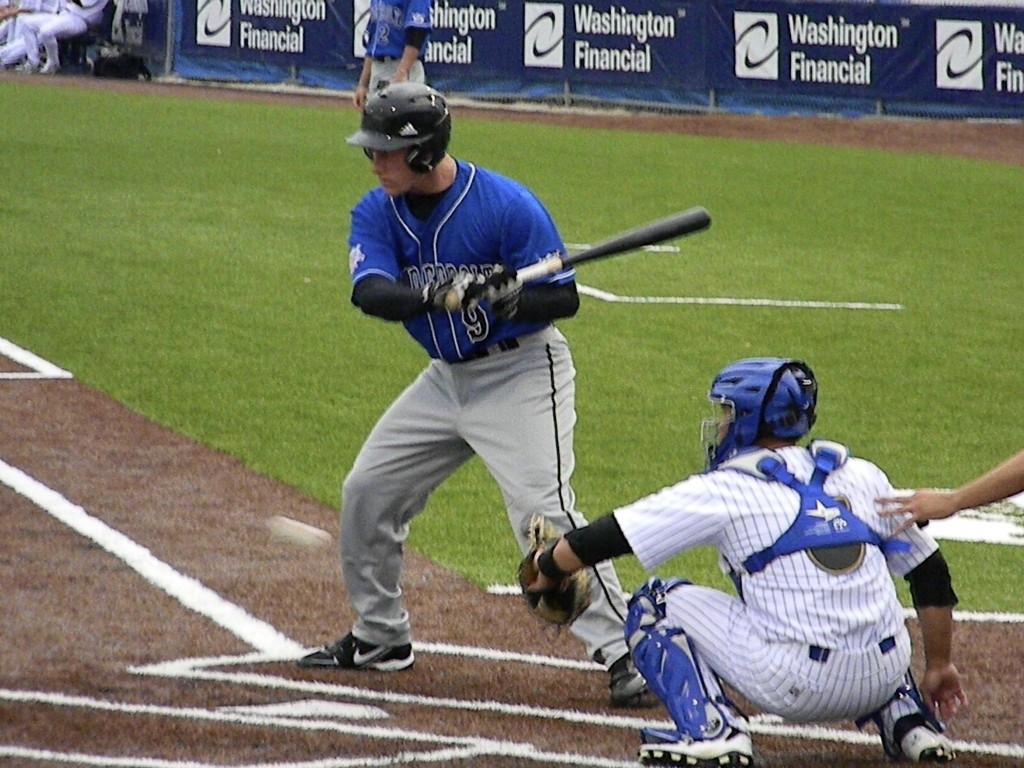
[514,512,595,634]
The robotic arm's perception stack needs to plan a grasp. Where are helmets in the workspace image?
[347,82,452,172]
[701,357,818,474]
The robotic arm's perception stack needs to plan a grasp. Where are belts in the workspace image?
[469,337,519,359]
[809,636,896,662]
[375,56,395,62]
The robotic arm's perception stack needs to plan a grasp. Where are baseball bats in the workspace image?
[444,204,715,310]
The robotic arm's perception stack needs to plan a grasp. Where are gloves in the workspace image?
[423,272,485,313]
[487,264,523,321]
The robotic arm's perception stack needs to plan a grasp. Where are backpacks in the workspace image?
[93,53,151,80]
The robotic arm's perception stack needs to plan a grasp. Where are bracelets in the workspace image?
[538,539,572,579]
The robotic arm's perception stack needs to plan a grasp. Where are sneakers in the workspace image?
[608,652,650,703]
[901,725,959,764]
[637,723,755,768]
[298,632,415,672]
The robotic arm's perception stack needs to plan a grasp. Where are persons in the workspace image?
[0,0,109,74]
[352,0,434,110]
[518,357,1024,768]
[299,83,660,705]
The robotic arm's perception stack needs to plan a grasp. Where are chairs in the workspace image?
[59,0,117,76]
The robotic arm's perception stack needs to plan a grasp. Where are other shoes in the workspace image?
[15,61,43,72]
[39,62,61,75]
